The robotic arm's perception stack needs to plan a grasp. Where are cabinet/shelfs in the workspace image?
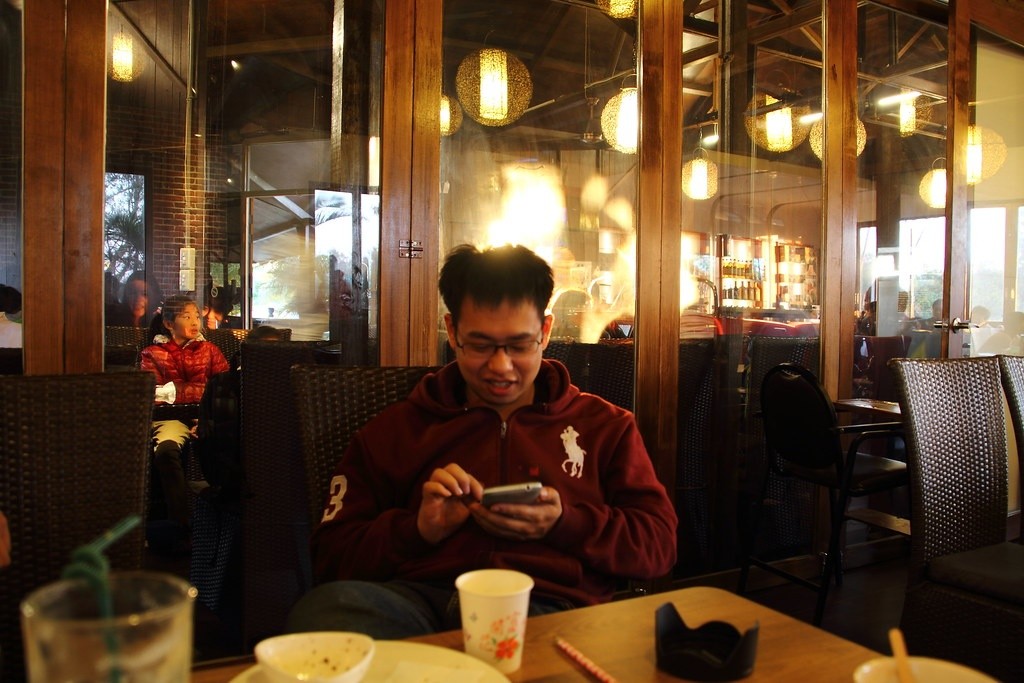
[775,241,820,308]
[716,234,765,319]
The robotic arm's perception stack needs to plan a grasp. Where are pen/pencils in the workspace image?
[555,637,617,683]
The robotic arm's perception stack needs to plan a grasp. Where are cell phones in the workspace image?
[480,482,543,509]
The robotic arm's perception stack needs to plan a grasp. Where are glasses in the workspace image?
[453,317,545,355]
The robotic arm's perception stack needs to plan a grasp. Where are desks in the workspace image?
[189,587,889,683]
[717,305,810,321]
[831,395,910,593]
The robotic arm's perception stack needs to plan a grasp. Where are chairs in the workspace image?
[0,329,1024,683]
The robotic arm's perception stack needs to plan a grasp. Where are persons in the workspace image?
[103,271,234,368]
[142,297,282,516]
[284,245,679,645]
[857,297,1024,357]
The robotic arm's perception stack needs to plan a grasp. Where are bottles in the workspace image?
[722,258,761,280]
[722,281,761,302]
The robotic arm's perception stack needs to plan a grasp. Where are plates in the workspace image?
[227,638,513,683]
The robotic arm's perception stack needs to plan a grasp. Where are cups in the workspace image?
[20,571,197,683]
[455,568,535,675]
[853,655,999,683]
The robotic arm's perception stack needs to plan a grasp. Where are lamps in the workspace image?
[107,23,143,80]
[439,0,1007,209]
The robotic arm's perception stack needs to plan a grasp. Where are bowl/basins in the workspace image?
[254,632,377,683]
